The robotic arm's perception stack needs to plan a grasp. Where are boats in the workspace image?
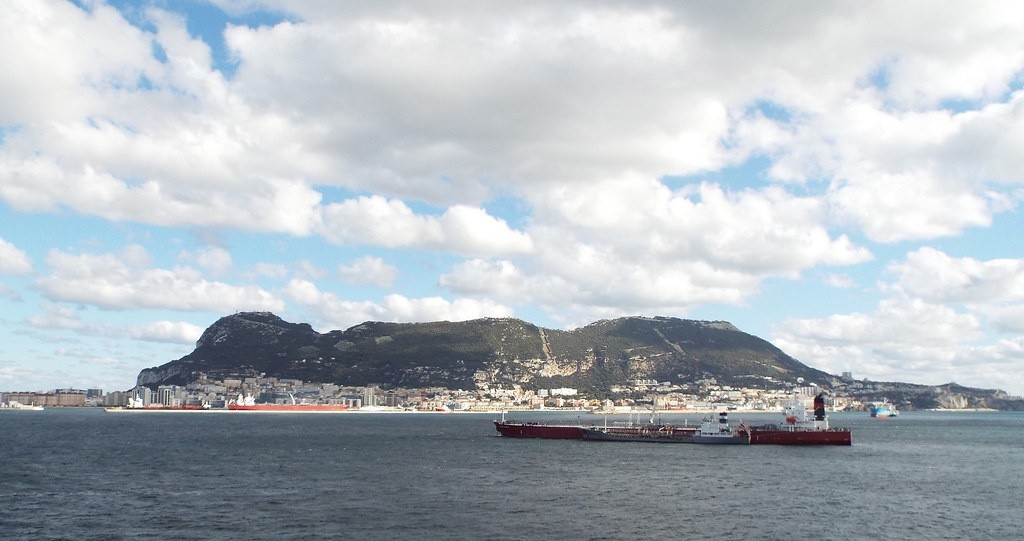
[871,406,899,418]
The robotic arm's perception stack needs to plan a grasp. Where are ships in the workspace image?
[492,395,852,447]
[228,402,349,410]
